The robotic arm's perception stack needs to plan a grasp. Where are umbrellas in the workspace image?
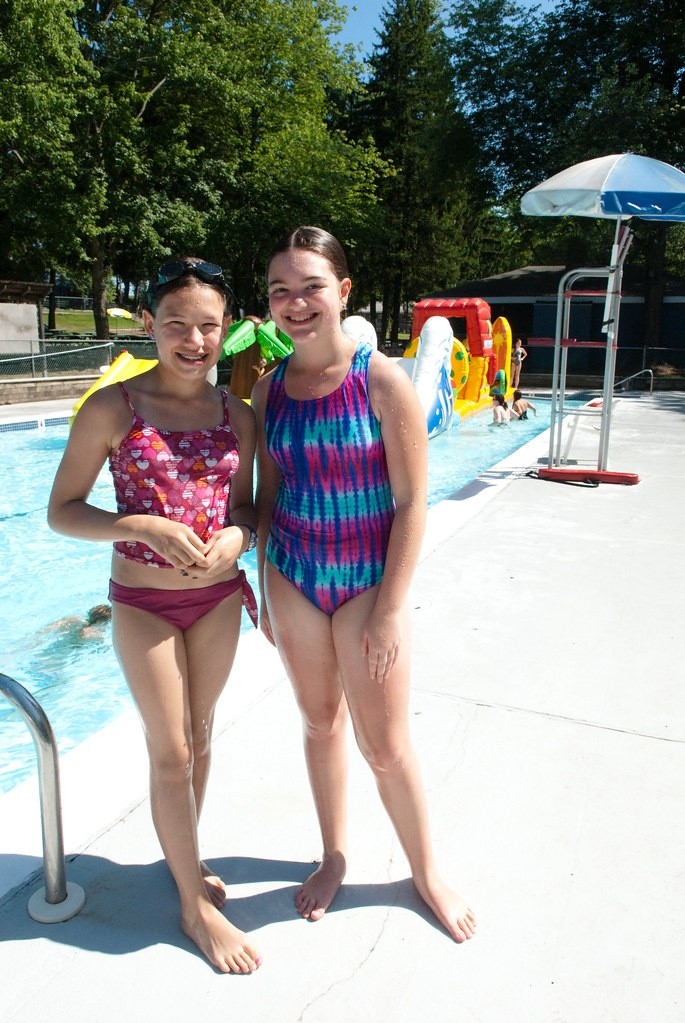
[106,308,132,319]
[520,153,685,335]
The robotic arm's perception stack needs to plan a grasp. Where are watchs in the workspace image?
[238,524,258,552]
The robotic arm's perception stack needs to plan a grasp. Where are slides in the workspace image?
[75,350,157,423]
[340,316,453,440]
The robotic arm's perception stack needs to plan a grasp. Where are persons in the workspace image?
[488,394,519,430]
[511,339,527,389]
[46,255,262,975]
[378,343,388,357]
[512,390,539,420]
[249,226,477,943]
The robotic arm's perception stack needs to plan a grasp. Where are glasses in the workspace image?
[147,261,239,312]
